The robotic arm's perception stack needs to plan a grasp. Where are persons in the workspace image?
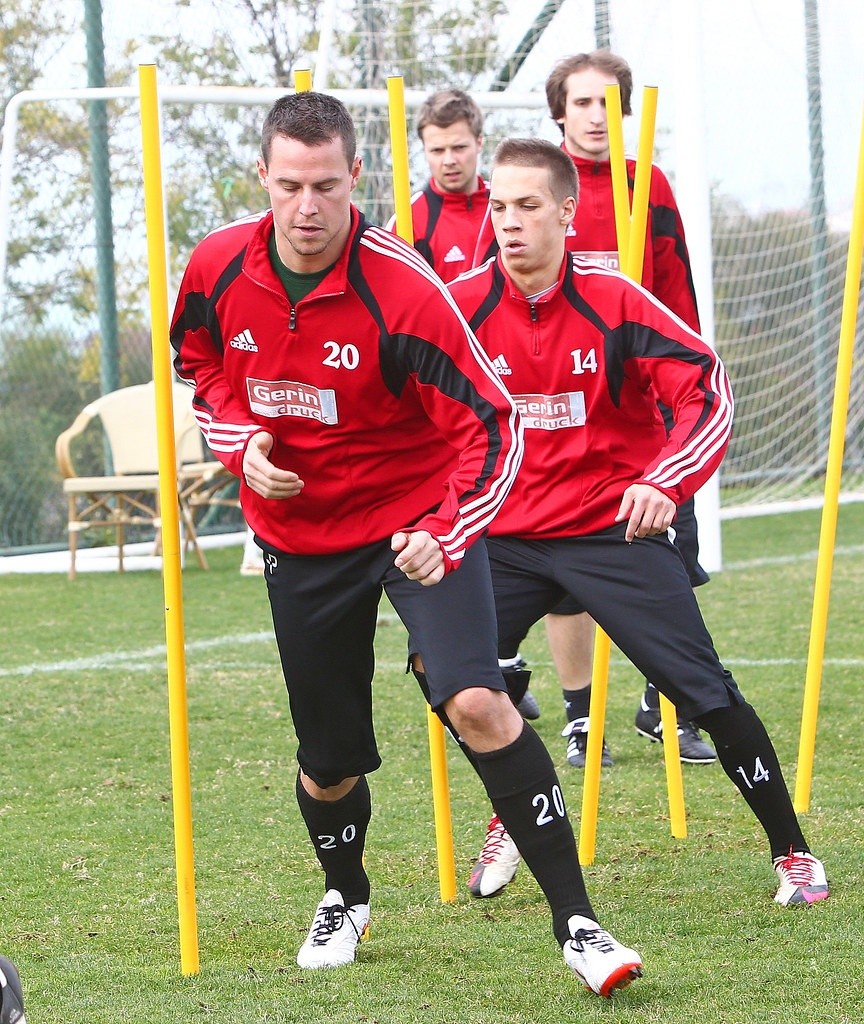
[407,140,829,905]
[385,54,717,768]
[171,91,643,997]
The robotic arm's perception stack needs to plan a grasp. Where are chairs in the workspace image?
[55,374,210,573]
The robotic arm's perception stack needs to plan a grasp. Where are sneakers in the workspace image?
[560,717,614,767]
[496,652,540,720]
[467,812,522,899]
[561,914,644,999]
[296,889,371,970]
[633,683,718,763]
[771,843,829,907]
